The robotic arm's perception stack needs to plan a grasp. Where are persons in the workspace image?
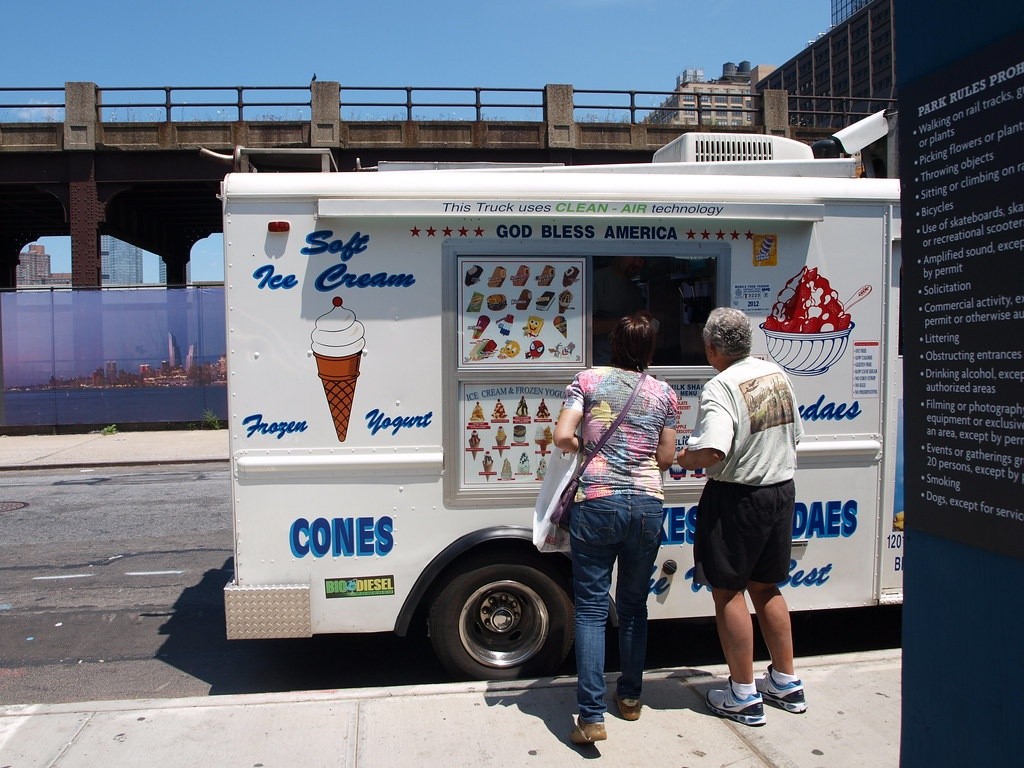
[677,306,809,726]
[552,310,678,744]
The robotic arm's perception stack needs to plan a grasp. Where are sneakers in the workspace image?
[705,675,767,725]
[754,664,808,713]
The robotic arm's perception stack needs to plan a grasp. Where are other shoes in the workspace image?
[613,691,641,720]
[570,715,607,744]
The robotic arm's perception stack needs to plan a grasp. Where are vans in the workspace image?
[216,107,905,680]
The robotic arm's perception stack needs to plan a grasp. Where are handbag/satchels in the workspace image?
[550,475,580,535]
[533,434,583,553]
